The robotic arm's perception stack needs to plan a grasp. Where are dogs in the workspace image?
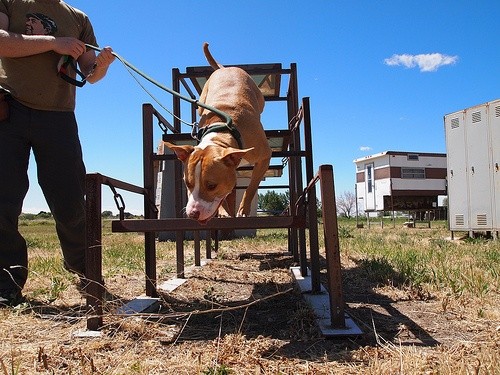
[162,42,273,226]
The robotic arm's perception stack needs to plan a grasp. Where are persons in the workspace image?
[0,0,114,303]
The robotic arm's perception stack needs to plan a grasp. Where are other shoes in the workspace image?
[63,262,105,296]
